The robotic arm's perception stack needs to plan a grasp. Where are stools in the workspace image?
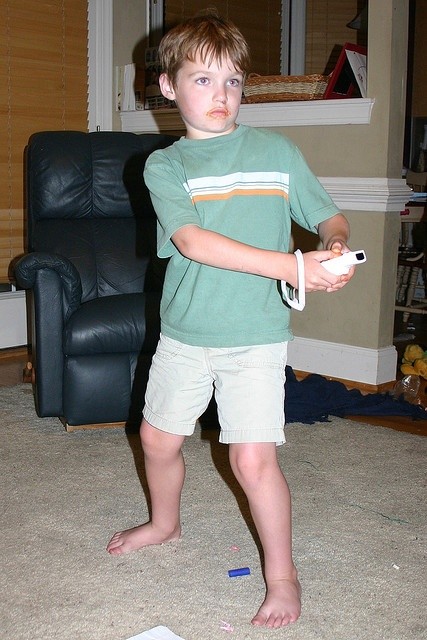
[397,255,427,324]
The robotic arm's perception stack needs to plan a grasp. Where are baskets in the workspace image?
[242,71,331,102]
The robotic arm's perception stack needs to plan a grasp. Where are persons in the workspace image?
[106,8,355,629]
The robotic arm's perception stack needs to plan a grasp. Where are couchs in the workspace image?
[7,130,180,433]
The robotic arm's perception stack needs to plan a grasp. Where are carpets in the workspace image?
[0,384,427,640]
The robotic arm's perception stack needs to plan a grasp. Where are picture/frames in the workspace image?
[323,41,368,100]
